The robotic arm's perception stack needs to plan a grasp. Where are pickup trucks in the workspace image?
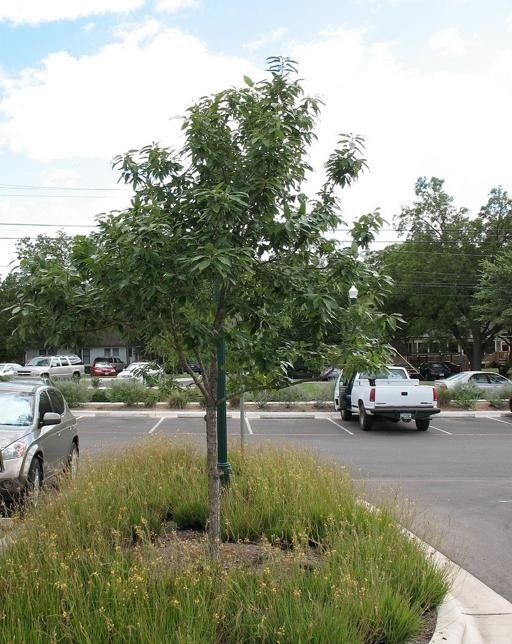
[333,366,441,433]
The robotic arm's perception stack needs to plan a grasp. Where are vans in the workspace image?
[14,355,85,380]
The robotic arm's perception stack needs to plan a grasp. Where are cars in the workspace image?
[418,360,461,379]
[0,377,79,518]
[115,361,166,380]
[433,370,512,398]
[90,357,124,377]
[166,355,201,374]
[0,362,22,380]
